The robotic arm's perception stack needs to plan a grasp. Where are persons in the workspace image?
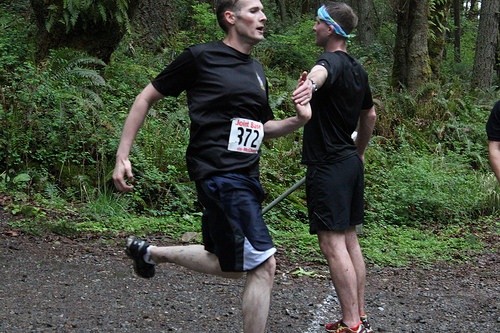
[113,0,312,332]
[485,99,499,180]
[292,0,374,333]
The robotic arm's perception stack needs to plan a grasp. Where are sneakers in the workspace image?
[325,315,373,333]
[328,321,367,333]
[125,237,155,279]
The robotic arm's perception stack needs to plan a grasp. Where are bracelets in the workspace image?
[308,76,318,93]
